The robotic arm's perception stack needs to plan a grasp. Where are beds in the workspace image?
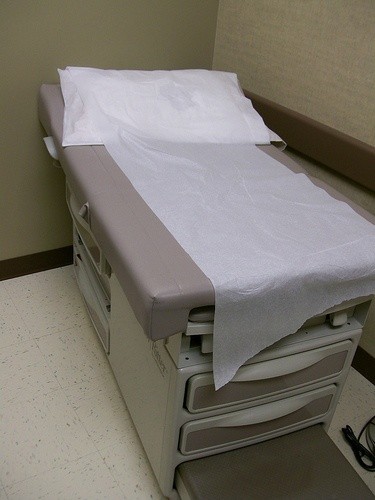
[35,81,375,500]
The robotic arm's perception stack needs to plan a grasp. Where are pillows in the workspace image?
[58,64,288,152]
